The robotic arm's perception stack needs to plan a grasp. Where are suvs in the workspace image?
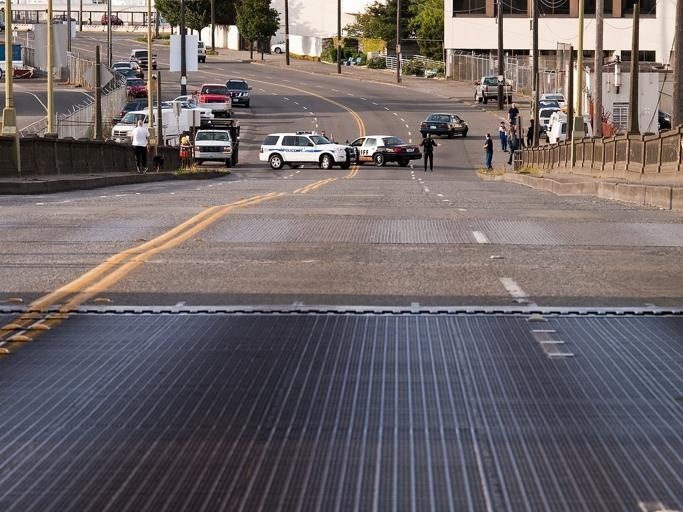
[101,15,122,25]
[258,130,355,170]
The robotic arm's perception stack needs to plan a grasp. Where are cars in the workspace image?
[534,90,594,147]
[657,111,672,133]
[271,41,286,53]
[52,14,75,24]
[344,134,422,166]
[110,41,252,169]
[419,112,468,138]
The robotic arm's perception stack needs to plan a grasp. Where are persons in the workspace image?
[508,103,519,127]
[499,119,542,166]
[316,131,329,144]
[345,140,349,146]
[483,133,493,170]
[418,134,442,172]
[180,134,193,170]
[131,120,150,173]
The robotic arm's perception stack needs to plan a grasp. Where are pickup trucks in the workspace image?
[473,76,512,104]
